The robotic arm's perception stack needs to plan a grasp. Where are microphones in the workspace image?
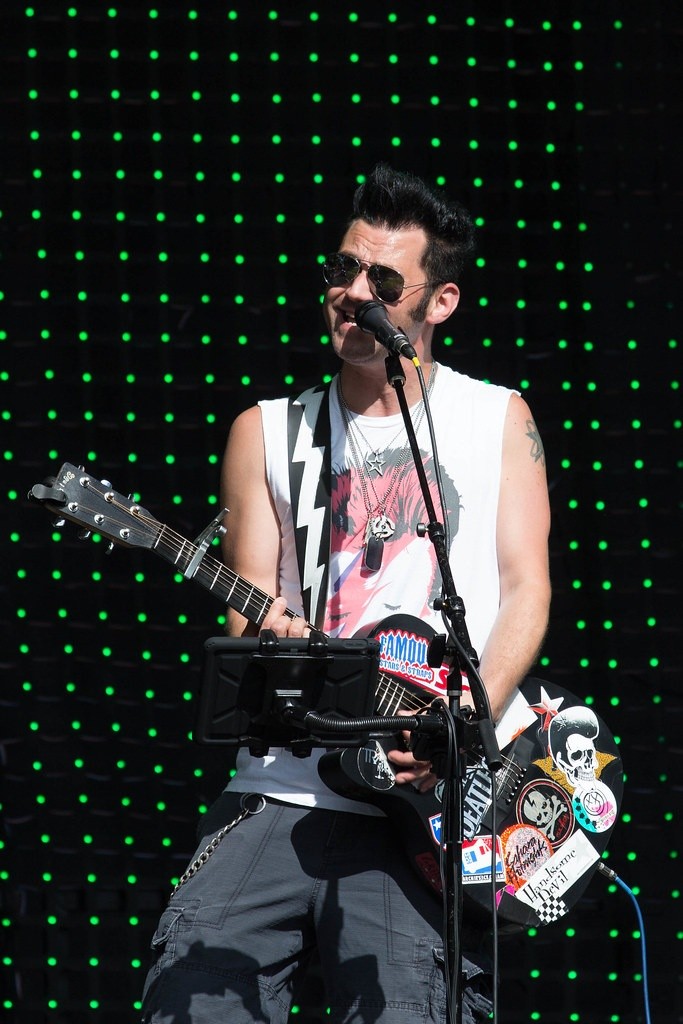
[354,298,421,367]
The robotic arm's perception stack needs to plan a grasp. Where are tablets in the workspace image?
[197,638,381,749]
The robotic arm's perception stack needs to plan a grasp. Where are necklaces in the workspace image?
[336,355,439,571]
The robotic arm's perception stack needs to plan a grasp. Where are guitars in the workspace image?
[24,463,626,931]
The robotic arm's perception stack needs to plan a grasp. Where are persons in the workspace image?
[137,164,553,1024]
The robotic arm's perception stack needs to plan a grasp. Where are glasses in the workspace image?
[322,251,446,305]
